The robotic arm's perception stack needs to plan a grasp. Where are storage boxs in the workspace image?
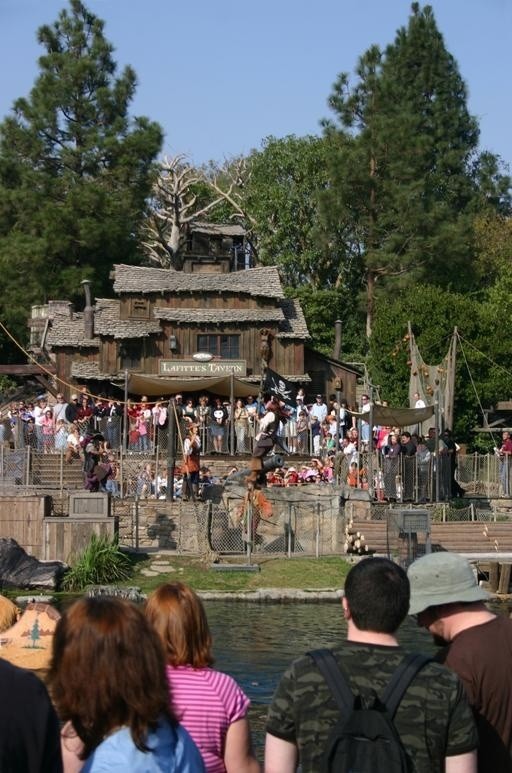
[67,491,112,518]
[384,507,431,534]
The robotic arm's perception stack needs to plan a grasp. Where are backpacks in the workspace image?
[305,648,430,773]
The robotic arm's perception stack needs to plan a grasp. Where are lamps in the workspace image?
[168,334,178,351]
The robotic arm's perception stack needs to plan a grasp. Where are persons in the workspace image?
[107,462,239,499]
[497,432,511,495]
[145,579,263,773]
[1,390,426,462]
[84,454,117,491]
[268,426,466,504]
[46,595,204,773]
[407,551,512,772]
[0,655,63,773]
[264,557,479,773]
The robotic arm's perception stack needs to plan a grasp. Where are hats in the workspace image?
[407,552,490,615]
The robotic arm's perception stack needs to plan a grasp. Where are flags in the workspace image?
[262,367,301,409]
